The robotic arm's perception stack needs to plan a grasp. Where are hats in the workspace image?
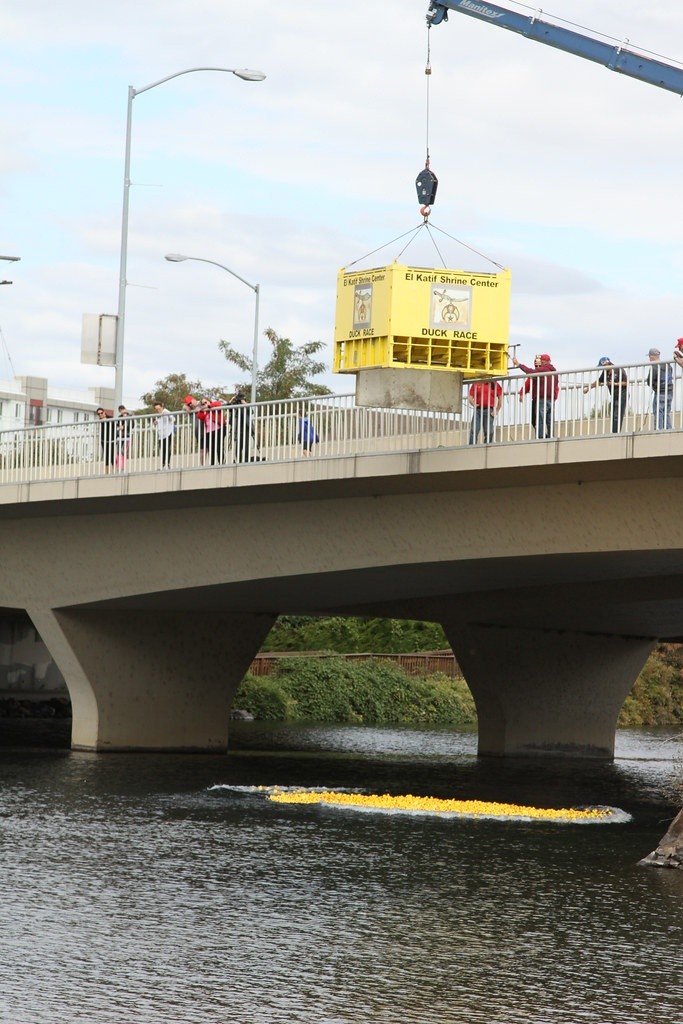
[185,396,192,404]
[645,348,660,355]
[540,354,550,360]
[596,357,610,366]
[675,338,683,348]
[236,393,246,400]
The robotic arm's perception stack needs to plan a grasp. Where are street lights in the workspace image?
[161,252,262,457]
[108,68,270,417]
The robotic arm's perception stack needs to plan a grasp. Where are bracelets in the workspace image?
[674,357,677,359]
[614,382,618,386]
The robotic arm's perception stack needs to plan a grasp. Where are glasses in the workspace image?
[535,364,541,368]
[201,401,207,406]
[98,411,104,415]
[187,402,192,405]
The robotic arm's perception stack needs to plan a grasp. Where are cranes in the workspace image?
[415,0,683,220]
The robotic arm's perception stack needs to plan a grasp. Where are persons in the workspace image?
[468,381,503,441]
[580,358,628,434]
[645,338,683,430]
[513,354,559,439]
[94,395,321,474]
